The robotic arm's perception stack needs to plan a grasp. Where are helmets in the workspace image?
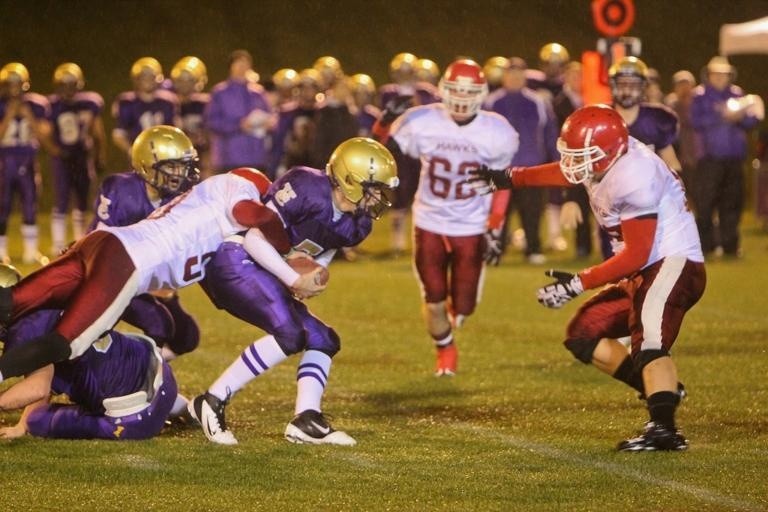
[0,61,31,96]
[536,41,571,78]
[324,136,400,222]
[553,102,630,186]
[169,55,209,97]
[130,123,201,197]
[269,54,377,102]
[482,54,510,87]
[387,51,441,85]
[129,56,165,92]
[604,55,649,109]
[51,61,85,93]
[436,58,489,118]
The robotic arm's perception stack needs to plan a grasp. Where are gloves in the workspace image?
[465,164,514,197]
[534,268,587,309]
[479,227,505,266]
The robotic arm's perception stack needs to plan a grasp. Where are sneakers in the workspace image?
[184,389,241,446]
[614,420,689,453]
[432,336,459,379]
[21,250,50,267]
[282,407,358,448]
[676,380,688,401]
[0,254,14,267]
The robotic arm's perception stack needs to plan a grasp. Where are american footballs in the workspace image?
[284,258,330,294]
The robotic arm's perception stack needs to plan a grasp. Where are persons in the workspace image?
[370,56,520,381]
[85,125,201,362]
[0,164,318,378]
[186,135,402,449]
[462,103,709,455]
[2,265,196,443]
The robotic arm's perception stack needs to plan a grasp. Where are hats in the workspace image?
[670,68,696,86]
[705,55,735,74]
[505,55,528,71]
